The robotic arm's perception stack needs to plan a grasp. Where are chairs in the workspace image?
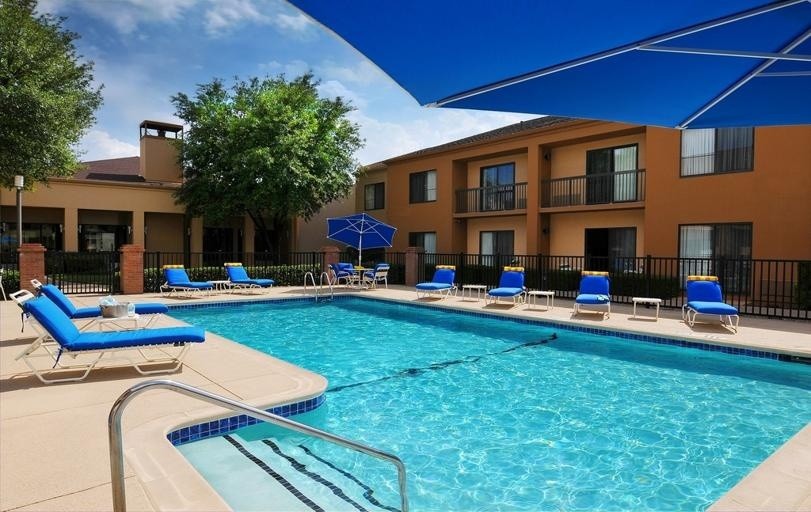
[159,264,213,297]
[363,264,390,289]
[415,265,458,301]
[224,262,274,293]
[573,271,611,319]
[488,266,527,308]
[682,275,739,335]
[30,278,168,331]
[9,289,205,383]
[328,264,350,285]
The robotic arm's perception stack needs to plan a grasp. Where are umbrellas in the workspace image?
[289,0,811,128]
[325,212,397,264]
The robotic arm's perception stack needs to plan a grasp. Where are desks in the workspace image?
[344,268,374,287]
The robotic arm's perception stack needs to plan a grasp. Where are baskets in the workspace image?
[99,297,129,317]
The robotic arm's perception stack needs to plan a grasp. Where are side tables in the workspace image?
[528,291,555,310]
[463,286,487,302]
[99,315,139,331]
[632,297,661,320]
[207,280,231,295]
